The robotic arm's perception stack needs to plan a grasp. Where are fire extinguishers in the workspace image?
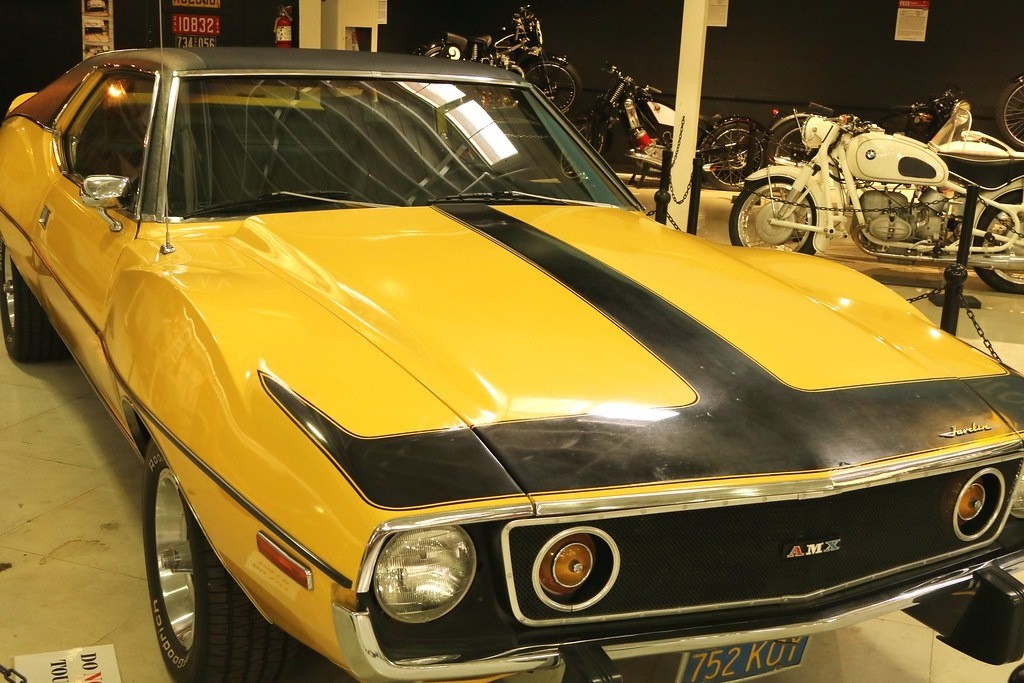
[273,4,293,48]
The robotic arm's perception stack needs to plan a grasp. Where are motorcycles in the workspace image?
[415,4,585,122]
[727,78,1024,294]
[556,62,774,191]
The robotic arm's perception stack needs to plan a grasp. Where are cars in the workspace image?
[89,46,110,54]
[0,46,1024,683]
[87,1,106,11]
[85,19,105,31]
